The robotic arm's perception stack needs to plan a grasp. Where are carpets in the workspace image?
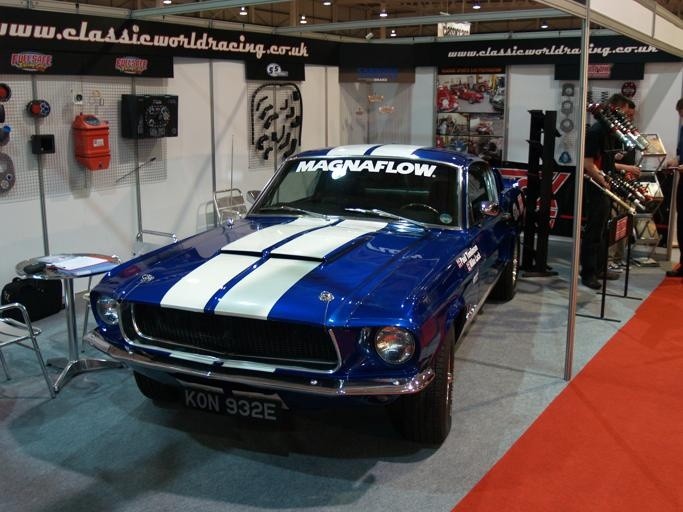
[451,262,682,510]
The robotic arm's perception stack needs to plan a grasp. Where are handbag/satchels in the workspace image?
[0,275,64,327]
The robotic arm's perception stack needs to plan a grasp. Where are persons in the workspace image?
[582,92,629,289]
[615,100,641,176]
[666,97,682,277]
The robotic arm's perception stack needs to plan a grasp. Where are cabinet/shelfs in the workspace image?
[632,132,667,268]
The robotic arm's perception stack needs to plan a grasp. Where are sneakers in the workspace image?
[617,260,632,270]
[581,276,601,290]
[608,263,624,273]
[666,266,683,278]
[598,271,618,280]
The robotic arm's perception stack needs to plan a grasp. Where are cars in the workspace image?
[437,113,493,136]
[451,84,484,104]
[490,87,503,110]
[80,142,526,449]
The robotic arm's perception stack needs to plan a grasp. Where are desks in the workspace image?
[16,252,125,394]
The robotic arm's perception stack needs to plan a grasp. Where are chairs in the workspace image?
[1,303,56,401]
[79,228,178,349]
[212,188,247,225]
[426,176,454,216]
